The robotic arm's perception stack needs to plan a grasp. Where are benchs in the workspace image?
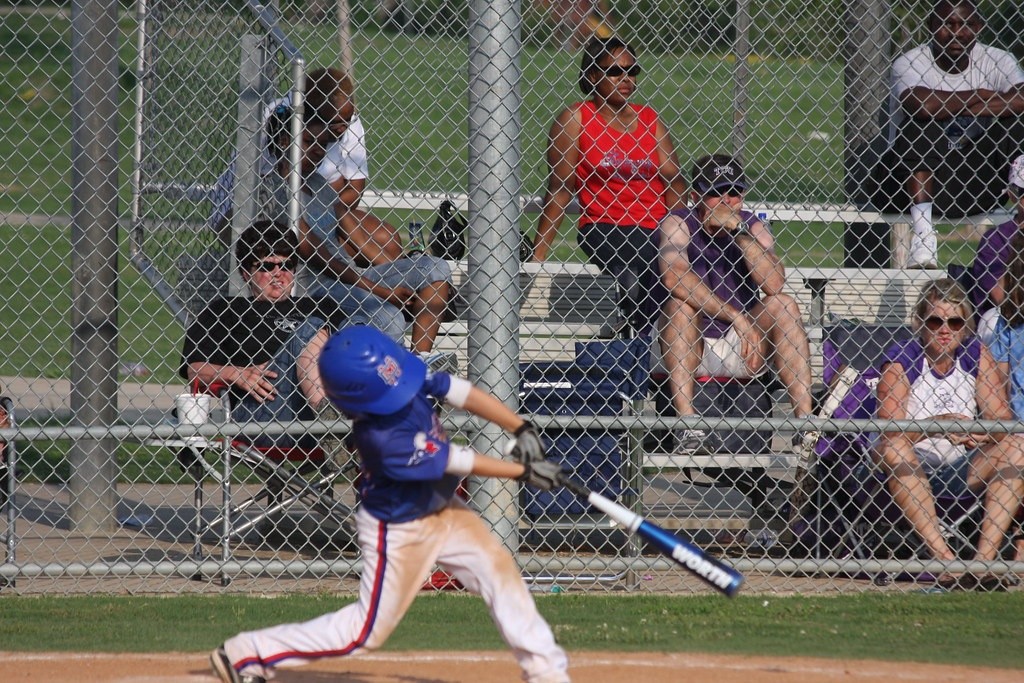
[355,189,1019,551]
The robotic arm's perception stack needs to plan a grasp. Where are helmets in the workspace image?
[318,325,428,418]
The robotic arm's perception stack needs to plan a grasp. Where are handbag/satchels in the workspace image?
[403,250,457,321]
[520,231,536,262]
[427,199,469,259]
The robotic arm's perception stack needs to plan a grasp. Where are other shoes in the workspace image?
[937,573,977,590]
[317,397,362,474]
[414,350,457,375]
[978,575,1007,592]
[621,313,638,336]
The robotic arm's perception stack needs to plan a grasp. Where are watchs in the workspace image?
[730,221,750,238]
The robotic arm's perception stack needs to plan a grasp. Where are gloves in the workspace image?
[509,420,547,463]
[513,459,564,489]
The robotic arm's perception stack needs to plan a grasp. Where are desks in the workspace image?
[125,438,222,581]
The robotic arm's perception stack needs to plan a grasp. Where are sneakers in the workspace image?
[793,429,821,457]
[210,642,267,683]
[673,414,715,453]
[906,228,939,268]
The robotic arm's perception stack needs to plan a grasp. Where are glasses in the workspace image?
[919,316,968,330]
[706,185,743,200]
[592,64,641,78]
[253,259,295,273]
[1009,184,1024,199]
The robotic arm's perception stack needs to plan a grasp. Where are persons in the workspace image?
[649,155,818,459]
[867,260,1024,593]
[210,326,570,683]
[887,0,1024,267]
[971,156,1024,314]
[179,220,359,476]
[214,68,459,375]
[531,39,687,332]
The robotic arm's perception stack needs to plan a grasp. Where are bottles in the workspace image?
[947,115,963,150]
[758,213,772,238]
[408,223,427,258]
[744,530,779,548]
[429,200,465,260]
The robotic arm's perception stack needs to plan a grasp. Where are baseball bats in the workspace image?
[503,438,745,599]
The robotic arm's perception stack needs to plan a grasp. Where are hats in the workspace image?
[1008,154,1024,188]
[692,155,746,195]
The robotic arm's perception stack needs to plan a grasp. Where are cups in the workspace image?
[174,394,210,442]
